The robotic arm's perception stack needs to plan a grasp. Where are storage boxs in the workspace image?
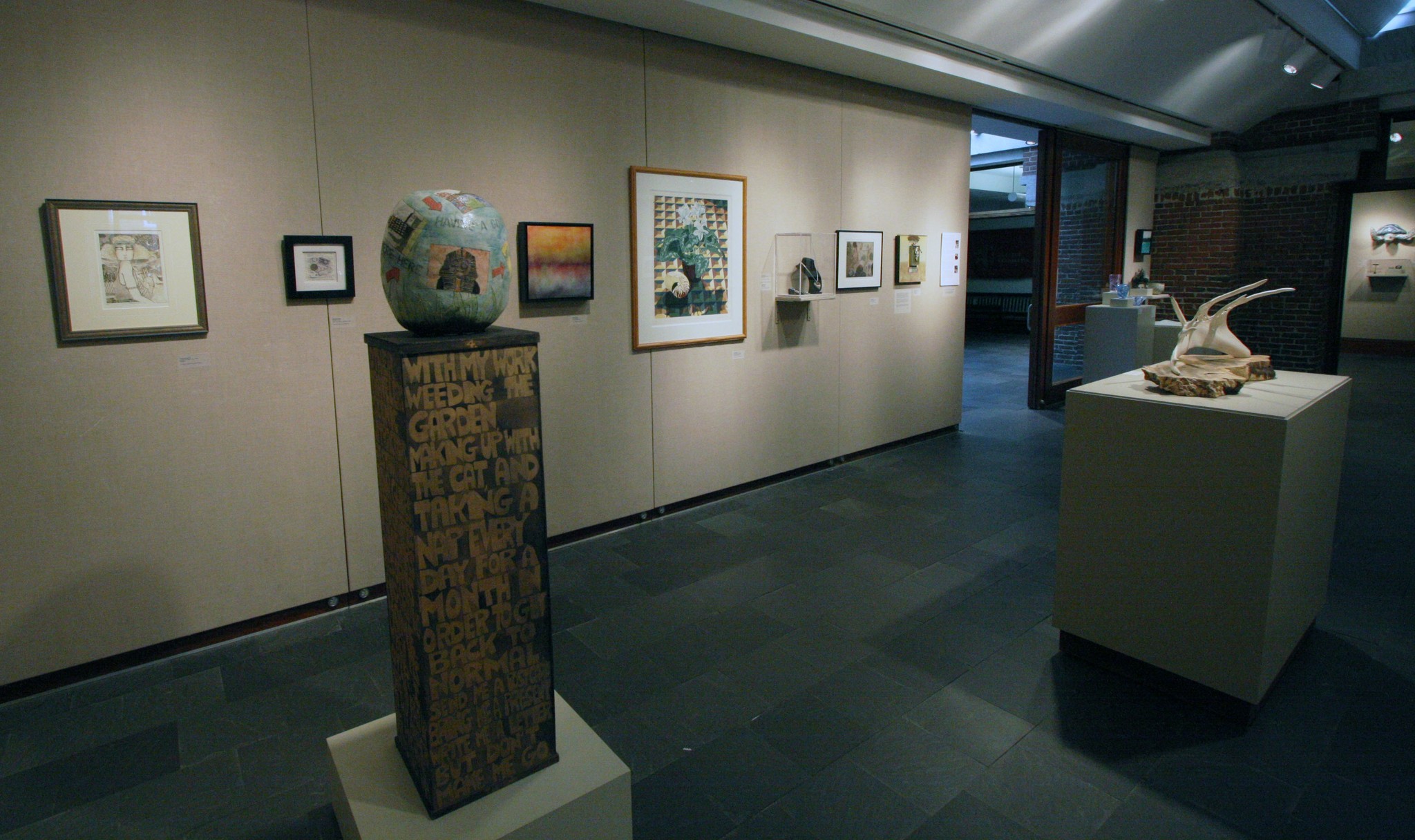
[775,231,837,302]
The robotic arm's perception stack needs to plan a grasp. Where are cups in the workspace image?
[1109,274,1122,292]
[1116,283,1130,299]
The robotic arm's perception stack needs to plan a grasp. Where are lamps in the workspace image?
[1008,166,1027,202]
[1388,116,1404,142]
[1280,35,1319,76]
[1310,55,1342,89]
[1256,13,1290,63]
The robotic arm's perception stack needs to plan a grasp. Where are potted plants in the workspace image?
[1147,280,1166,295]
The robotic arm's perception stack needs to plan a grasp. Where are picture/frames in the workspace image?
[835,229,884,291]
[517,221,595,303]
[282,234,356,300]
[629,165,748,352]
[44,197,209,344]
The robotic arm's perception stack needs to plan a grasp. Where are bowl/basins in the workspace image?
[1128,294,1146,305]
